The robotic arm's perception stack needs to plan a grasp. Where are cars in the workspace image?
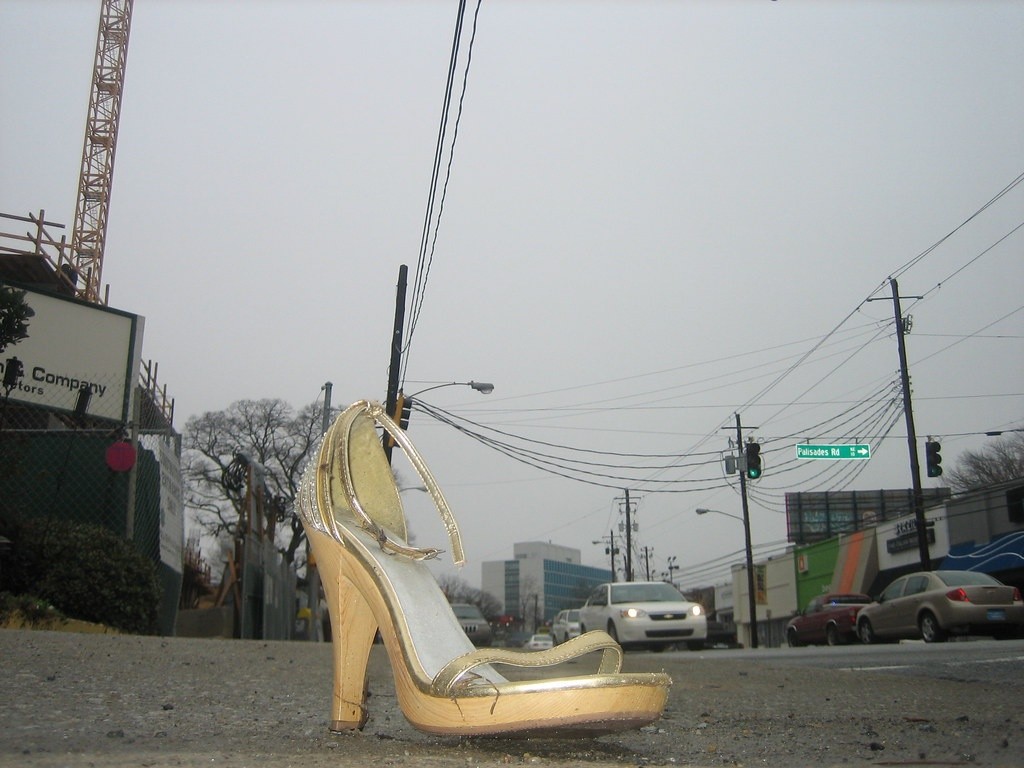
[785,592,875,646]
[856,570,1024,644]
[527,635,554,651]
[448,603,492,647]
[551,608,583,645]
[579,582,708,654]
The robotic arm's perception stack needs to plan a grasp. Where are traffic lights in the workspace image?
[926,441,945,476]
[745,442,764,481]
[388,391,414,453]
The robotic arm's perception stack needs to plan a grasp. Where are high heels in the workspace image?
[292,400,672,740]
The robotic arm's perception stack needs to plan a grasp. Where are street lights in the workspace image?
[592,539,616,582]
[697,507,758,649]
[378,380,495,477]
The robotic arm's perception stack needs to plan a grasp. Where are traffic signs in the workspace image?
[797,442,871,460]
